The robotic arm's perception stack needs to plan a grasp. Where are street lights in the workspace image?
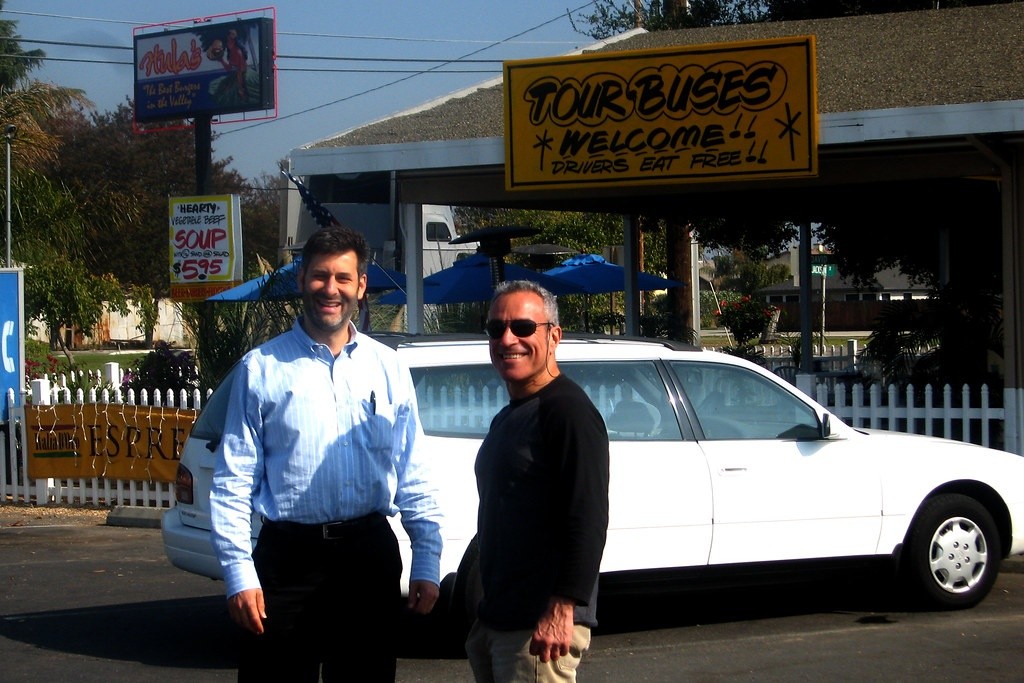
[5,124,15,267]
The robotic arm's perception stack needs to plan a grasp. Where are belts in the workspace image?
[263,513,385,541]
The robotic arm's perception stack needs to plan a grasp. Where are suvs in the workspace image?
[160,324,1023,654]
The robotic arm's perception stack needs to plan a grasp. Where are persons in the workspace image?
[464,280,609,683]
[206,255,408,300]
[208,228,443,683]
[376,250,584,331]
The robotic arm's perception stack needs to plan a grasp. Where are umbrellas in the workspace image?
[541,252,689,334]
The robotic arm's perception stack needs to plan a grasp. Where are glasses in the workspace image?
[483,317,556,339]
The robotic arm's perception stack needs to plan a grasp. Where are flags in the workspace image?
[288,174,339,228]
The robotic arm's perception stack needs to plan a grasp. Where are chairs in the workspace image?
[607,400,662,439]
